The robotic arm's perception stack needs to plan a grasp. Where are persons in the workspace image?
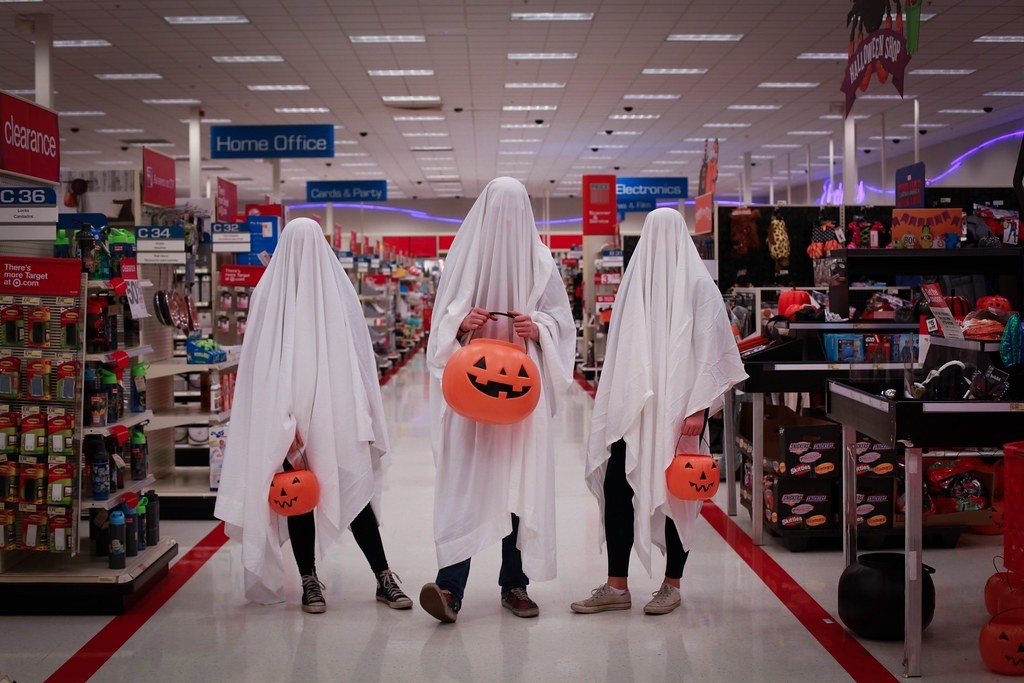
[214,217,414,613]
[420,177,576,624]
[571,207,750,616]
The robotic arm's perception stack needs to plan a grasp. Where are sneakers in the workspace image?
[419,583,462,622]
[376,570,413,608]
[501,586,539,616]
[570,584,632,613]
[644,583,681,613]
[299,569,327,611]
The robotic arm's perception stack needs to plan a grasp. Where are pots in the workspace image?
[153,264,170,326]
[170,264,200,330]
[161,264,177,328]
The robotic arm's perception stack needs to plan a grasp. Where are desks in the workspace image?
[825,379,1024,677]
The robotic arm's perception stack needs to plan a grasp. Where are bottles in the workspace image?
[135,497,148,551]
[86,298,118,354]
[144,489,159,546]
[124,428,146,481]
[205,369,223,415]
[83,435,125,501]
[130,361,150,413]
[84,368,124,427]
[108,511,126,570]
[73,223,95,280]
[53,229,70,258]
[120,295,140,347]
[90,228,136,280]
[122,504,138,556]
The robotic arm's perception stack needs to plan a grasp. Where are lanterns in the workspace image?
[268,469,319,516]
[667,454,720,501]
[441,337,542,425]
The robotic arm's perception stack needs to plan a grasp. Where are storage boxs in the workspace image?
[731,335,995,551]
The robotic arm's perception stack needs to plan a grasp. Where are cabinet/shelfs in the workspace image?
[0,86,439,617]
[713,202,1024,545]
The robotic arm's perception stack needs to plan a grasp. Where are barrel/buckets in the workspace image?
[838,552,935,643]
